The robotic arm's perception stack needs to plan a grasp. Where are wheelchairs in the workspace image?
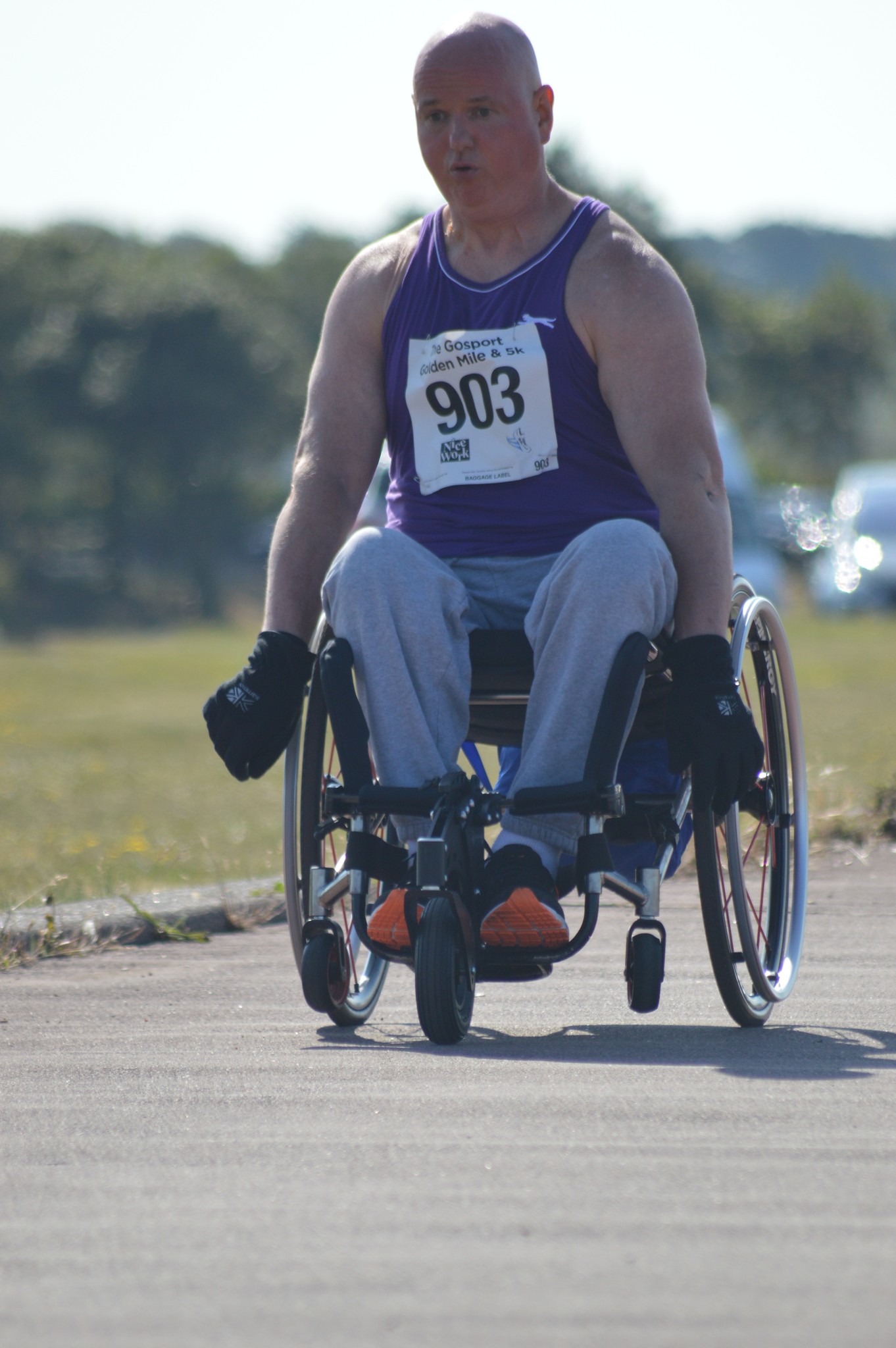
[281,572,810,1048]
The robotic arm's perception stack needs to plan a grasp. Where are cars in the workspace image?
[800,458,896,614]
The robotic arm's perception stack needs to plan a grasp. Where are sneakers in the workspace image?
[475,844,571,944]
[365,854,424,947]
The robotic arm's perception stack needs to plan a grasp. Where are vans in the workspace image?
[700,400,788,612]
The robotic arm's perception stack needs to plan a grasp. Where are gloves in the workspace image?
[670,635,765,815]
[203,631,317,783]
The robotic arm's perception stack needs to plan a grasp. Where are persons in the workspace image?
[202,10,762,981]
